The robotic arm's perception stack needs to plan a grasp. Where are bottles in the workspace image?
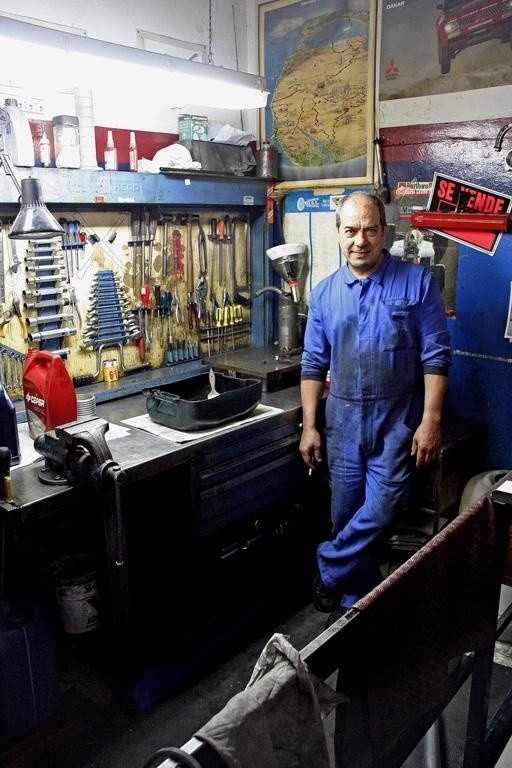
[129,131,138,171]
[104,129,118,170]
[39,130,50,167]
[261,142,271,149]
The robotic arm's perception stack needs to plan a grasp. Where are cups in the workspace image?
[102,361,118,381]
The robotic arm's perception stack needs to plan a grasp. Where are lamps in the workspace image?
[0,129,68,245]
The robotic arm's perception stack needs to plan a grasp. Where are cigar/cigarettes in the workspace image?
[308,456,315,476]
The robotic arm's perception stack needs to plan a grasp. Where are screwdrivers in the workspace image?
[215,306,242,351]
[141,286,174,347]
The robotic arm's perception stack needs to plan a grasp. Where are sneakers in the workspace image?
[312,557,341,613]
[325,606,349,630]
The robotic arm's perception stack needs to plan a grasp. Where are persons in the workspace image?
[297,191,452,629]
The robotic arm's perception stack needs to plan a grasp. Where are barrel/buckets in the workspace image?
[21,347,78,438]
